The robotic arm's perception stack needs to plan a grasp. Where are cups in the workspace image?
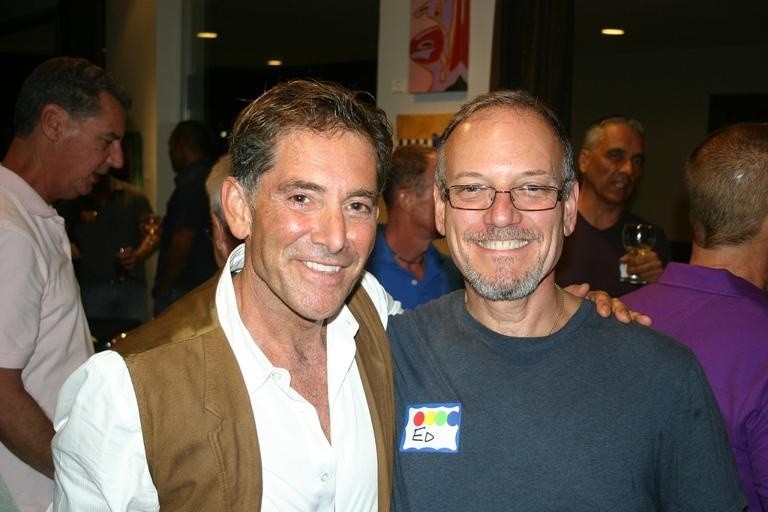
[443,179,568,211]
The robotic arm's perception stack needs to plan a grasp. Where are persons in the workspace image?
[554,113,670,297]
[52,175,160,354]
[50,77,652,511]
[363,144,465,311]
[385,90,750,510]
[613,121,766,512]
[152,120,219,319]
[4,57,132,512]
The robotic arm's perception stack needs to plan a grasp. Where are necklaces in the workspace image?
[393,250,424,264]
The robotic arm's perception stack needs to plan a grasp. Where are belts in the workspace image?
[143,214,161,245]
[116,248,129,282]
[620,222,659,284]
